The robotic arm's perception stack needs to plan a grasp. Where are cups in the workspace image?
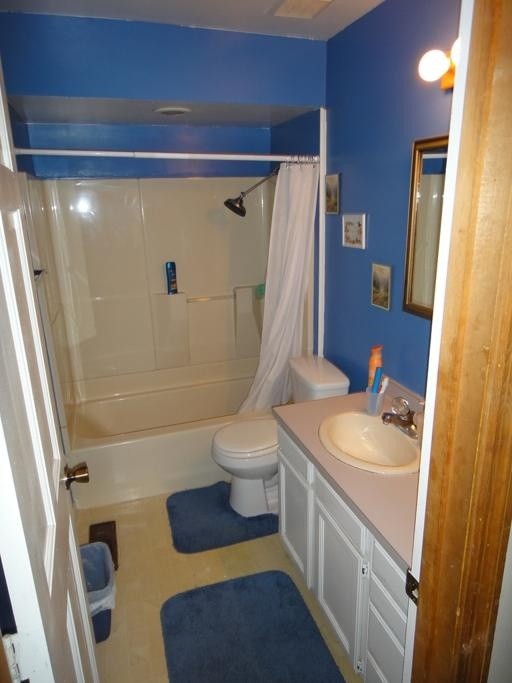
[366,386,385,417]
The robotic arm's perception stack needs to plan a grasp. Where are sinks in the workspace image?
[316,409,422,477]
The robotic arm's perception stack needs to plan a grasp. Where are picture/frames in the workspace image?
[323,173,392,313]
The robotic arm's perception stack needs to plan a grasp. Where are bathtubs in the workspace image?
[68,357,274,511]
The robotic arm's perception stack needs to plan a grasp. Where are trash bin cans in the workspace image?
[79,542,115,643]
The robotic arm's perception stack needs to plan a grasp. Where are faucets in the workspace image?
[380,394,419,441]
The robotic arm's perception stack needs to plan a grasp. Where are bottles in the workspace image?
[367,345,384,386]
[166,261,177,295]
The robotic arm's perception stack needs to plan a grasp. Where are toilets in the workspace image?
[211,354,351,518]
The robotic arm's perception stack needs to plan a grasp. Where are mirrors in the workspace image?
[402,137,448,319]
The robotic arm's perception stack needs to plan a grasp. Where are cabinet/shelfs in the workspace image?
[274,428,408,683]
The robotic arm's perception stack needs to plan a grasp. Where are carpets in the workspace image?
[162,478,277,553]
[157,572,347,682]
